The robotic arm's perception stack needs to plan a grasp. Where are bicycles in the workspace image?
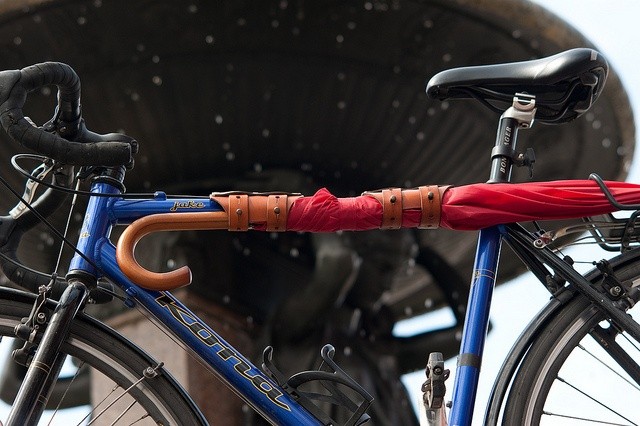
[0,47,640,426]
